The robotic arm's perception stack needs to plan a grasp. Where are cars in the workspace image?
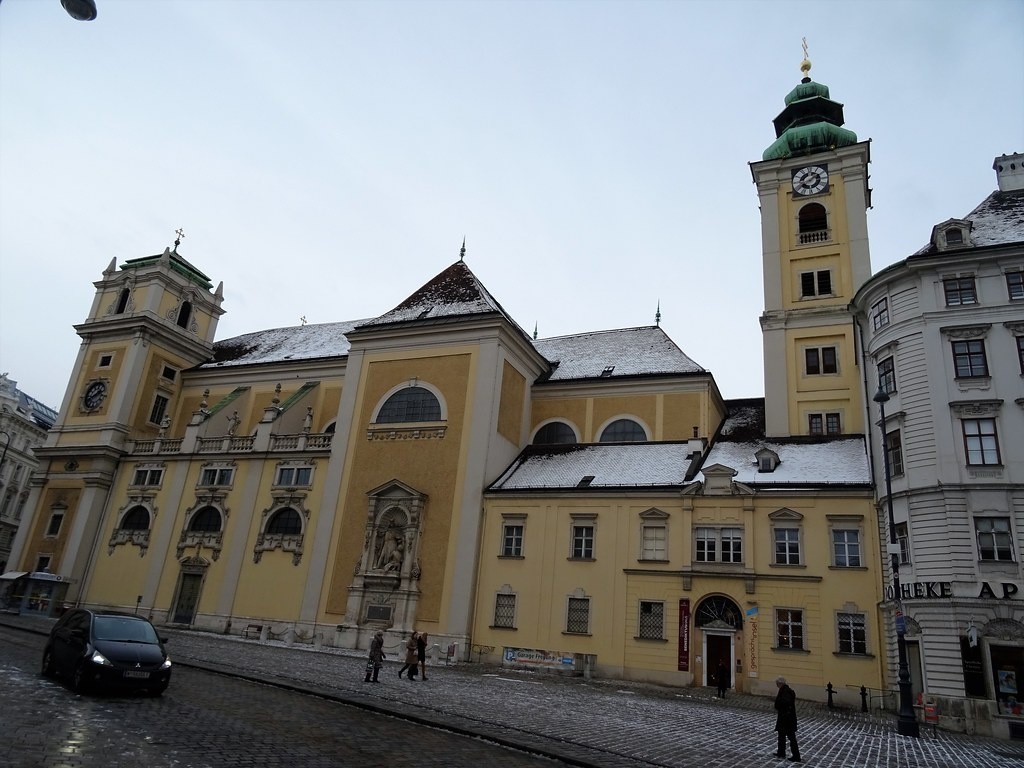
[40,608,172,700]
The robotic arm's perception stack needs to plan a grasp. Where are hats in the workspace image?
[776,676,786,683]
[377,630,383,634]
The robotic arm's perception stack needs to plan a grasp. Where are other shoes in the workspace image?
[398,671,401,678]
[773,751,786,756]
[788,756,801,762]
[408,676,415,680]
[422,676,428,679]
[374,678,379,683]
[722,694,724,698]
[365,679,372,682]
[718,694,720,697]
[409,666,418,675]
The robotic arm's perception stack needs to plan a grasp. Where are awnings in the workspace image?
[0,572,28,581]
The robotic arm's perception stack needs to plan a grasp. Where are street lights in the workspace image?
[871,381,922,738]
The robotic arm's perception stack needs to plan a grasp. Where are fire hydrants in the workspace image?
[860,684,868,713]
[827,681,834,711]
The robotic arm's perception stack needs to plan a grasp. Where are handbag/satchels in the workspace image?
[366,658,375,671]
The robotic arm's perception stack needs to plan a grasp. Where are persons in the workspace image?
[373,523,403,575]
[364,630,386,683]
[407,632,429,681]
[158,413,171,438]
[398,632,419,681]
[773,676,801,762]
[713,662,726,698]
[301,406,314,434]
[226,410,241,435]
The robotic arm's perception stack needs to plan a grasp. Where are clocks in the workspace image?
[790,162,830,199]
[84,382,106,408]
